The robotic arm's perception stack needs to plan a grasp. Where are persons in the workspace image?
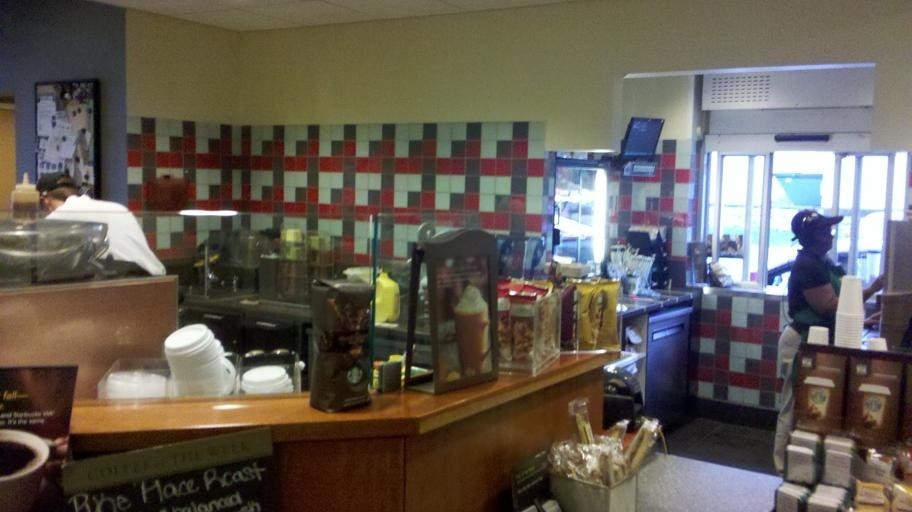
[771,209,886,478]
[37,171,169,277]
[706,233,744,259]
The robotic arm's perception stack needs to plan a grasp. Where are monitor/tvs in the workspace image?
[620,117,665,162]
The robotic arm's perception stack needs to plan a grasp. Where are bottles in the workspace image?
[9,172,41,219]
[373,361,383,390]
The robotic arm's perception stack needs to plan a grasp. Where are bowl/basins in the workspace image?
[0,219,111,283]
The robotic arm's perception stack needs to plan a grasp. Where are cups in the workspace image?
[858,382,891,431]
[804,377,836,421]
[0,429,70,512]
[806,274,889,354]
[454,304,495,379]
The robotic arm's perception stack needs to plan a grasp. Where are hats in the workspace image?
[36,172,76,195]
[791,210,843,241]
